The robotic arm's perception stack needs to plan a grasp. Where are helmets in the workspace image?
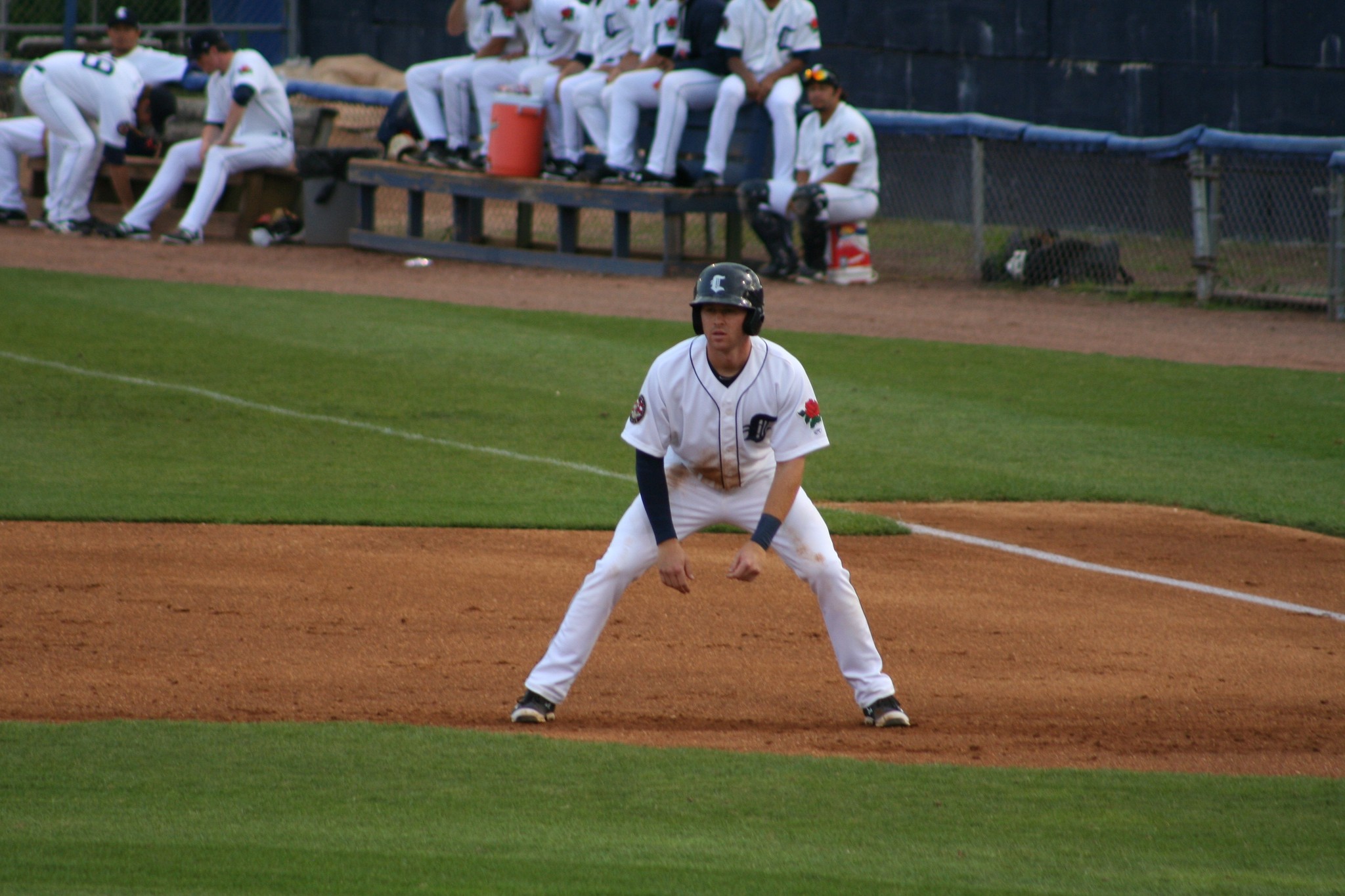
[689,262,764,335]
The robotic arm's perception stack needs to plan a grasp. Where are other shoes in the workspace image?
[401,139,720,188]
[796,268,826,284]
[0,207,27,224]
[46,214,128,238]
[762,262,798,278]
[160,228,201,246]
[116,221,150,240]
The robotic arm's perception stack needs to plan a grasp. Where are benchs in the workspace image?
[338,87,773,278]
[27,96,340,246]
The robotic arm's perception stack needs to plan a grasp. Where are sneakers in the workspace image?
[863,696,910,728]
[511,689,555,722]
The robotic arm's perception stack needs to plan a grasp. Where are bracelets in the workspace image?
[751,512,782,552]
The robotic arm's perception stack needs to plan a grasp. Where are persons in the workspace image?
[741,62,879,283]
[0,7,294,246]
[406,0,822,276]
[511,262,909,729]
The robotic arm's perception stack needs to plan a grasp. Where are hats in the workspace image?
[151,87,176,135]
[187,29,224,56]
[802,63,840,87]
[107,5,137,28]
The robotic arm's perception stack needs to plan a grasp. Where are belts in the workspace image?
[34,65,45,73]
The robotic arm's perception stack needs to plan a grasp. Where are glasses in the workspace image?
[801,69,839,86]
